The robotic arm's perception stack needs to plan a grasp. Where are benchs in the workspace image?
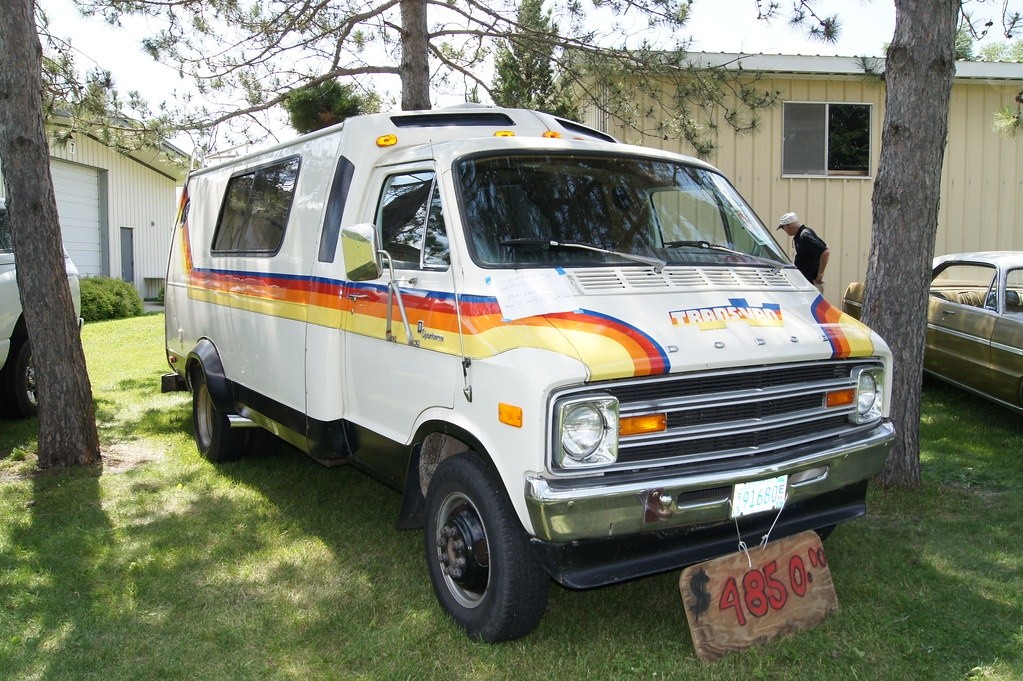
[933,290,1023,311]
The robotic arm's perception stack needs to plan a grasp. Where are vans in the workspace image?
[162,104,895,643]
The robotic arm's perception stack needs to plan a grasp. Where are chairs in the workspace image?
[957,290,1020,313]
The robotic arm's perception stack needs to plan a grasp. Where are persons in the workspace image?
[776,212,830,294]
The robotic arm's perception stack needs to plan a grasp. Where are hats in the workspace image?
[776,213,797,230]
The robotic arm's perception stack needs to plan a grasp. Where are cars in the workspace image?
[844,252,1023,420]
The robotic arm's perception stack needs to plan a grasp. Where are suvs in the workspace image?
[0,199,84,413]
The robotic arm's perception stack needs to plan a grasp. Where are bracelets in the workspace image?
[818,272,825,276]
